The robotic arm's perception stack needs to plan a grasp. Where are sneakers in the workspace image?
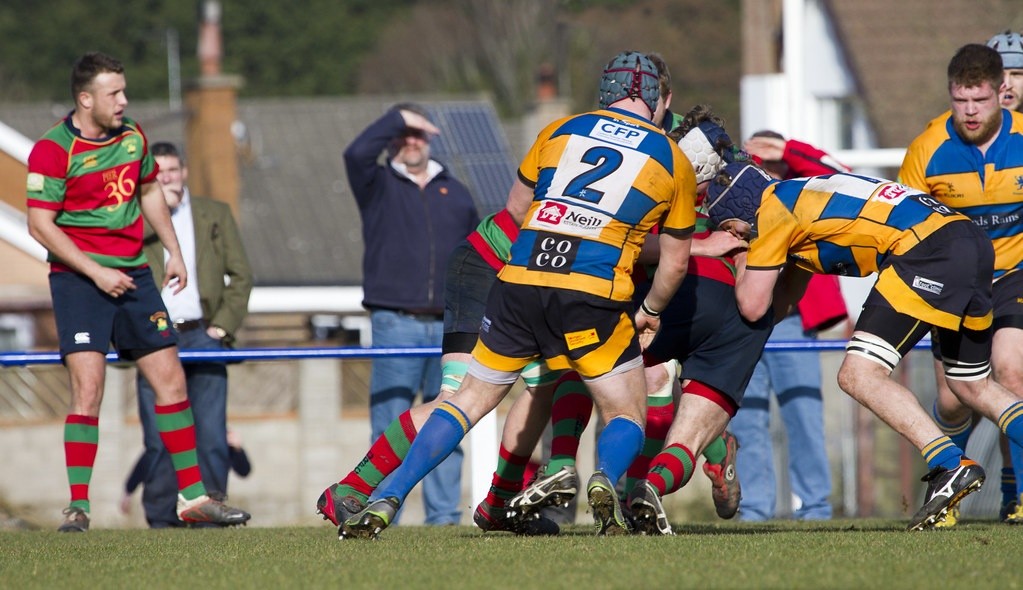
[588,471,628,536]
[700,430,741,521]
[1006,493,1023,524]
[630,480,676,534]
[57,507,89,533]
[506,466,577,518]
[472,498,561,535]
[337,496,399,540]
[906,455,986,532]
[177,493,250,527]
[315,482,366,525]
[935,504,959,528]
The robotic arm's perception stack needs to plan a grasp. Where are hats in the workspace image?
[679,120,734,184]
[705,161,773,229]
[988,31,1023,70]
[598,50,660,120]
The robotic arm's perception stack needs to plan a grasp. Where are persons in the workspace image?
[343,103,484,526]
[136,141,254,530]
[520,68,573,162]
[27,51,252,532]
[119,428,252,515]
[317,28,1023,540]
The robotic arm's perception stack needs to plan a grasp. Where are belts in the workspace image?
[174,318,200,333]
